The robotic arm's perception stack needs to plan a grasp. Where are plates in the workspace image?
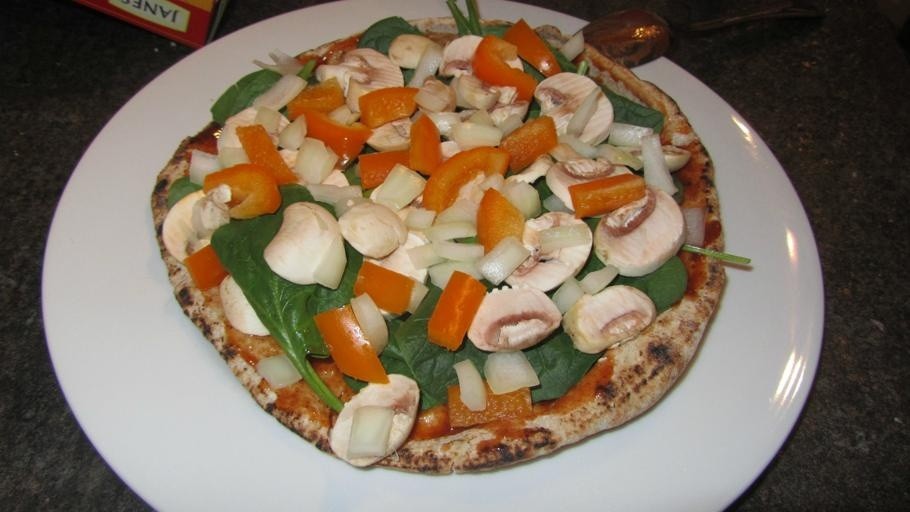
[36,0,828,512]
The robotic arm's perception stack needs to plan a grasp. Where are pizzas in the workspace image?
[151,2,751,476]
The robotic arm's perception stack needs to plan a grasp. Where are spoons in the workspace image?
[580,0,834,69]
